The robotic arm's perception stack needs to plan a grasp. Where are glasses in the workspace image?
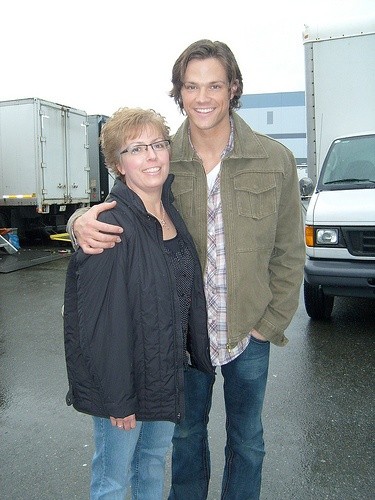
[120,141,171,155]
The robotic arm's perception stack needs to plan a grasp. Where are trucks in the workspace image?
[2,97,123,242]
[299,22,375,322]
